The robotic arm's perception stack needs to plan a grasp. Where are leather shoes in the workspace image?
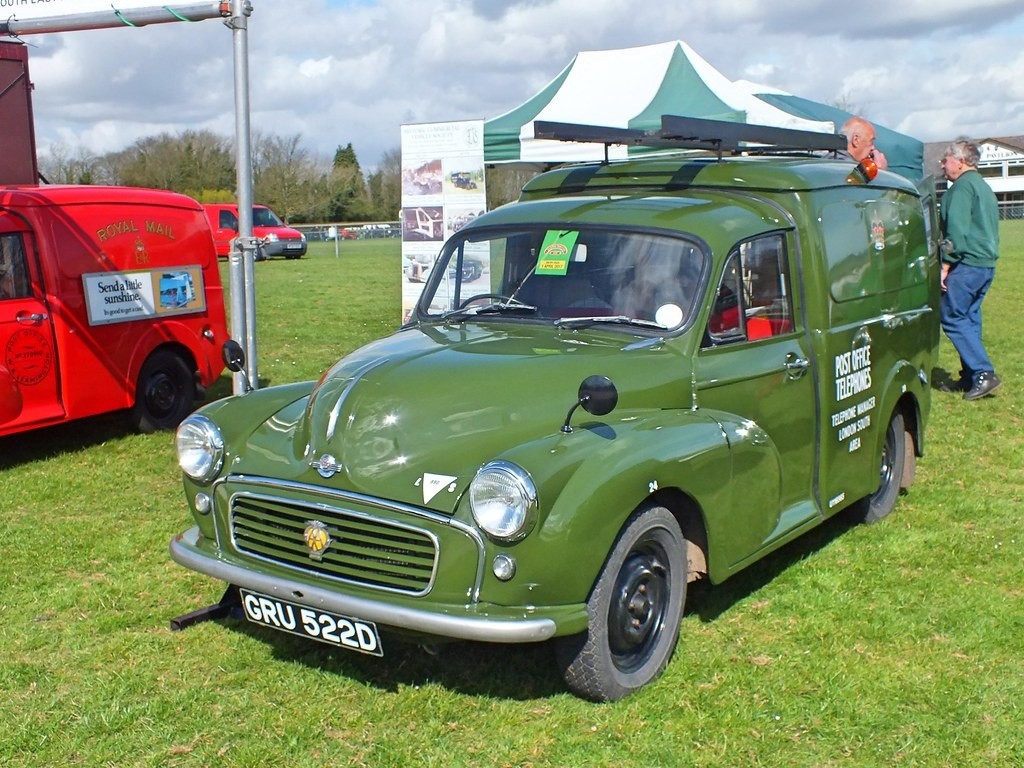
[940,369,1003,399]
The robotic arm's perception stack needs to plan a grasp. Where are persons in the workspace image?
[938,137,1003,400]
[819,116,889,173]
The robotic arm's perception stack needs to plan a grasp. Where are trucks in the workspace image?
[0,183,231,433]
[200,203,308,260]
[167,103,943,707]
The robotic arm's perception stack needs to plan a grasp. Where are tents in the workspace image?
[482,40,835,172]
[733,78,925,182]
